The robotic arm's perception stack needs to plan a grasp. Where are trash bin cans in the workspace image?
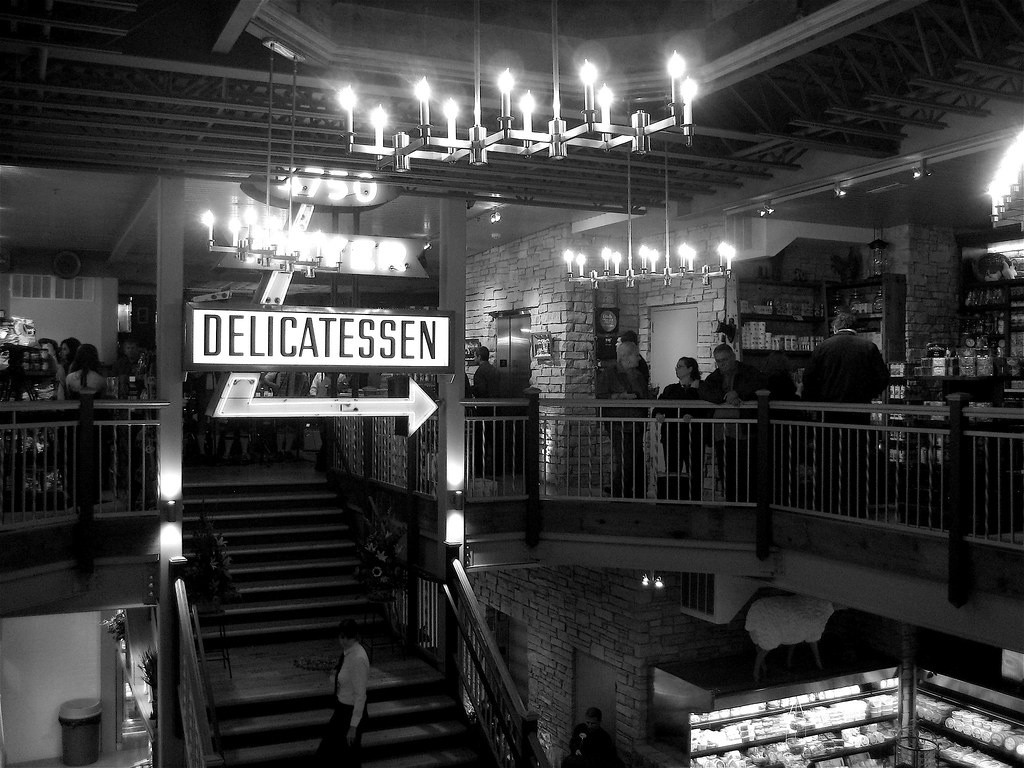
[58,698,104,765]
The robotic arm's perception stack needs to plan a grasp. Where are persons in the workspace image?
[702,342,775,508]
[115,338,148,495]
[561,706,618,768]
[310,618,371,768]
[185,372,351,472]
[593,330,661,499]
[653,356,705,502]
[801,313,893,518]
[38,337,106,502]
[465,346,502,481]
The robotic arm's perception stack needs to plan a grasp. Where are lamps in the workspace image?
[203,195,345,278]
[759,200,774,218]
[913,158,931,181]
[642,569,665,590]
[335,0,699,172]
[832,182,848,199]
[563,154,736,288]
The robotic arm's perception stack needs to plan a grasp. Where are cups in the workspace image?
[742,321,797,350]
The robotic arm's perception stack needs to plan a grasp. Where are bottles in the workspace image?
[798,336,824,350]
[848,289,864,313]
[830,288,844,315]
[872,286,882,313]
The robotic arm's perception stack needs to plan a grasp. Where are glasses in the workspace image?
[677,364,684,368]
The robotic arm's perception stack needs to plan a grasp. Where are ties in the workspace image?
[333,652,345,699]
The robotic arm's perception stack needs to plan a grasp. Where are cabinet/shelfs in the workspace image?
[647,617,1024,768]
[887,223,1023,463]
[732,236,906,366]
